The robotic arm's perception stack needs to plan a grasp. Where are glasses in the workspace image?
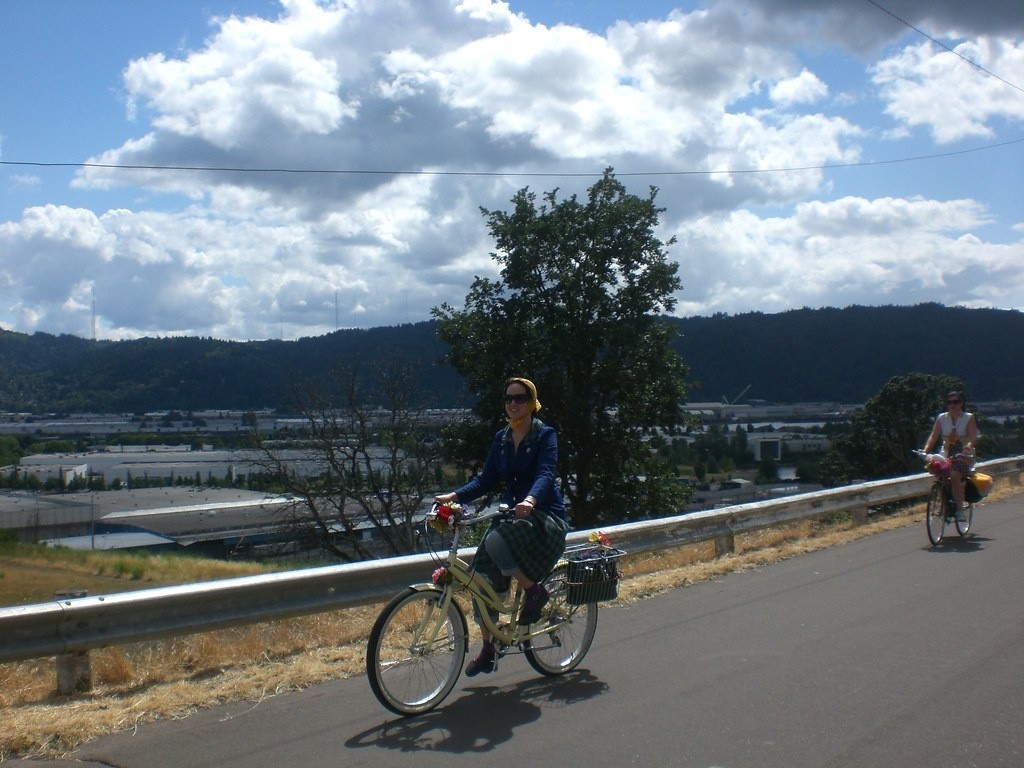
[945,399,960,405]
[502,394,530,404]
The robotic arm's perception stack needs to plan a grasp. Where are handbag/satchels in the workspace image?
[947,472,993,503]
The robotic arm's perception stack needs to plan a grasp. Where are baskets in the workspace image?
[552,544,627,609]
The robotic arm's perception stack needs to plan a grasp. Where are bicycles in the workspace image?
[912,449,974,545]
[366,501,626,718]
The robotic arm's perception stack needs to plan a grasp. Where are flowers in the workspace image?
[589,531,610,546]
[433,567,452,586]
[429,501,463,532]
[925,461,942,472]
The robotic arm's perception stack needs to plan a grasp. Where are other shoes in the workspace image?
[465,646,505,677]
[956,511,967,521]
[518,583,550,625]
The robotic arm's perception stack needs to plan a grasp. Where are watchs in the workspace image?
[525,498,534,505]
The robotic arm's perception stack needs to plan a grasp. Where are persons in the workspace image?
[434,378,570,677]
[917,392,977,521]
